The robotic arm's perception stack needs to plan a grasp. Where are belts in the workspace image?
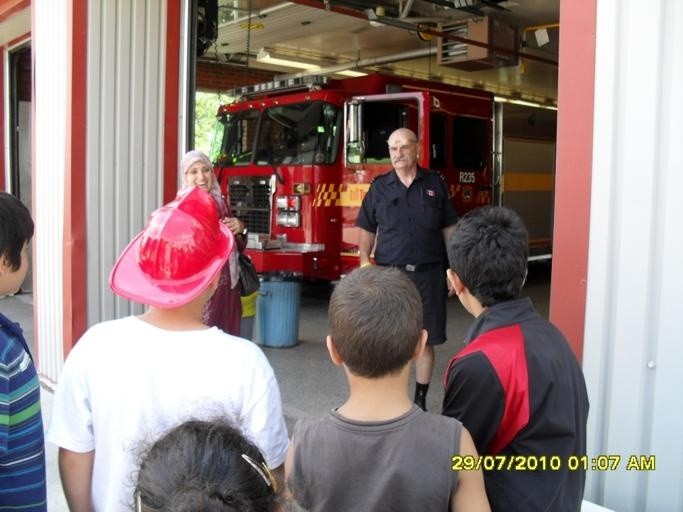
[378,264,438,271]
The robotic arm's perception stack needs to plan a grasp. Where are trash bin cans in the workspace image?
[256,270,303,349]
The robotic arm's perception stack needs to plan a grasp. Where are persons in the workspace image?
[48,186,288,512]
[0,191,47,511]
[441,203,589,511]
[173,150,248,338]
[353,129,458,412]
[284,264,493,510]
[126,416,284,510]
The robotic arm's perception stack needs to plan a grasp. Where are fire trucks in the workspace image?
[210,72,557,296]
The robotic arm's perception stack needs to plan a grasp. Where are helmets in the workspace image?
[110,186,234,308]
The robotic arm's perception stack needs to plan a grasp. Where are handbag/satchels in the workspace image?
[237,255,260,298]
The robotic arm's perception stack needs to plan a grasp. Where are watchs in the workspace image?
[237,229,246,240]
